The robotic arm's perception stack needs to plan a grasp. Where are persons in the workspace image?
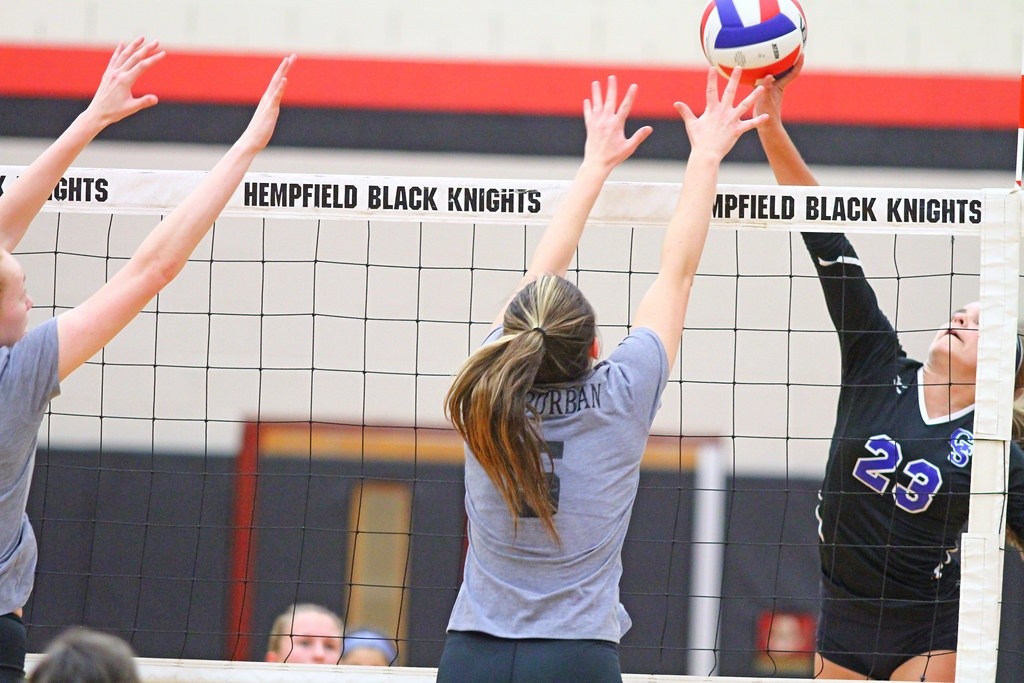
[751,52,1023,683]
[429,64,767,683]
[0,36,303,683]
[262,601,398,666]
[25,624,144,683]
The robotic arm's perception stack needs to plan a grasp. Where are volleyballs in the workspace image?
[699,0,807,86]
